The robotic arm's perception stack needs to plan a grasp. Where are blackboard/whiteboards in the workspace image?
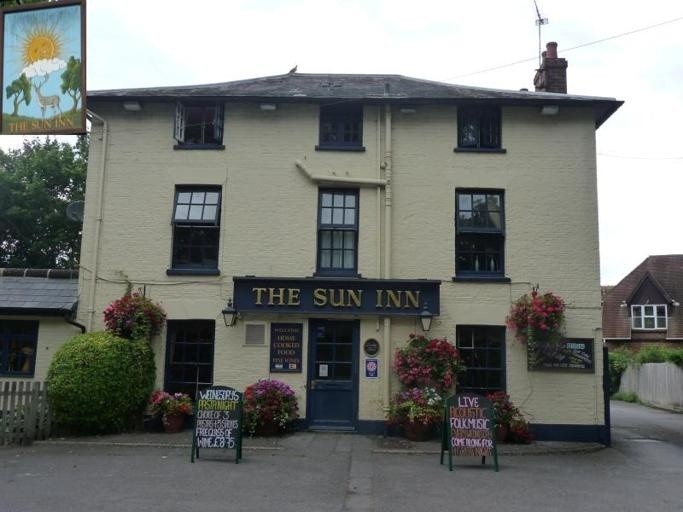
[445,392,497,456]
[192,386,242,449]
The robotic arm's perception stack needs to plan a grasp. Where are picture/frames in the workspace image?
[1,0,86,135]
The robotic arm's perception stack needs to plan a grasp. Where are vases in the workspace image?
[257,416,279,436]
[519,431,533,444]
[408,417,434,442]
[495,420,510,441]
[162,413,182,433]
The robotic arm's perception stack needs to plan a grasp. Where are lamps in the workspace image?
[420,303,432,331]
[222,298,237,326]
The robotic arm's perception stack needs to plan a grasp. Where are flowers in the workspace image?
[102,282,164,338]
[387,387,444,424]
[491,391,519,424]
[242,378,296,425]
[512,411,529,434]
[505,286,563,340]
[151,388,191,415]
[395,333,459,396]
